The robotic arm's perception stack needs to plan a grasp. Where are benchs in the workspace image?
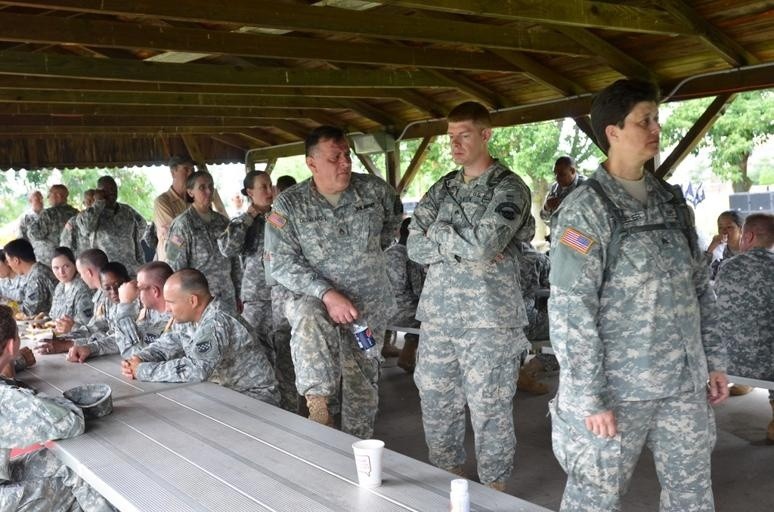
[383,321,550,354]
[540,343,774,395]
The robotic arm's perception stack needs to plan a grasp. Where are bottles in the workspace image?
[349,318,379,359]
[449,478,470,512]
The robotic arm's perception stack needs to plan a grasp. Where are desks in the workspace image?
[1,288,560,511]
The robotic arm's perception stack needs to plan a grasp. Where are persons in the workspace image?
[1,152,302,415]
[711,212,774,443]
[0,304,121,512]
[381,217,431,372]
[547,77,731,511]
[512,215,561,397]
[262,124,405,440]
[405,100,535,493]
[702,209,757,397]
[538,157,588,227]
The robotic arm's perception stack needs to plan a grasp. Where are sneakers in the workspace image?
[397,343,416,371]
[306,396,332,425]
[730,384,753,396]
[382,344,398,357]
[519,370,549,394]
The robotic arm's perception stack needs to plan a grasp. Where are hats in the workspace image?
[63,383,113,417]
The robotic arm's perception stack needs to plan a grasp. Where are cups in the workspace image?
[352,437,385,488]
